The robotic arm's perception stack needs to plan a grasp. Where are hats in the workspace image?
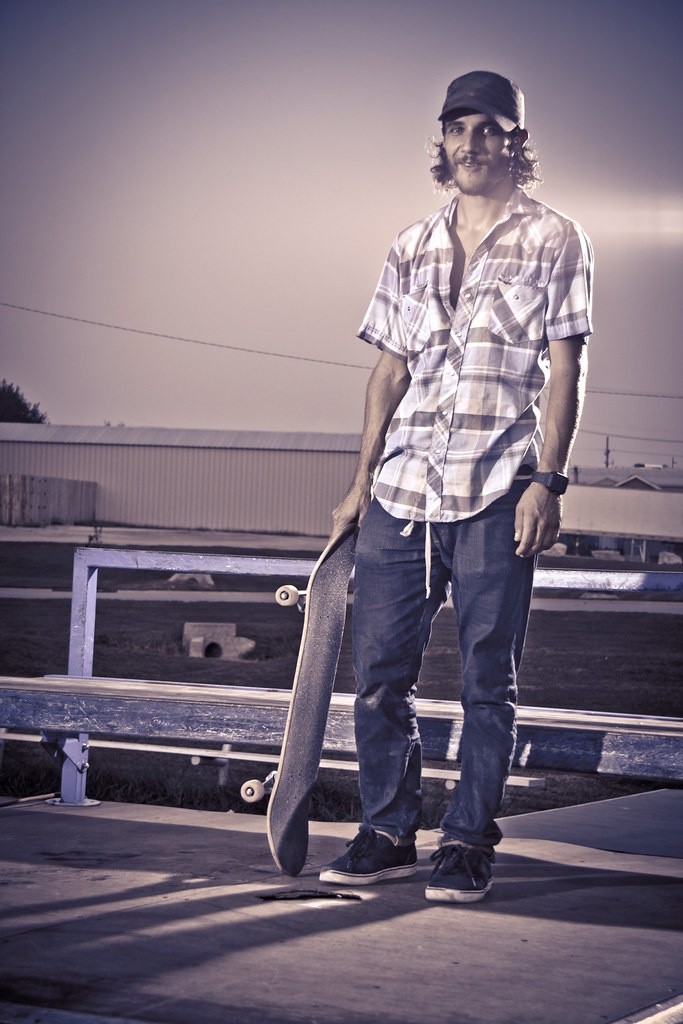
[438,70,525,132]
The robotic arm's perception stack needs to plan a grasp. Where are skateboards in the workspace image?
[238,522,360,876]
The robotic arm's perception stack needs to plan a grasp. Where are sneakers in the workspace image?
[319,829,418,885]
[425,838,493,902]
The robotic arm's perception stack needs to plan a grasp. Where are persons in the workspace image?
[318,66,595,903]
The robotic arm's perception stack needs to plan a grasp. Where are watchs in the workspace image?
[530,468,570,495]
